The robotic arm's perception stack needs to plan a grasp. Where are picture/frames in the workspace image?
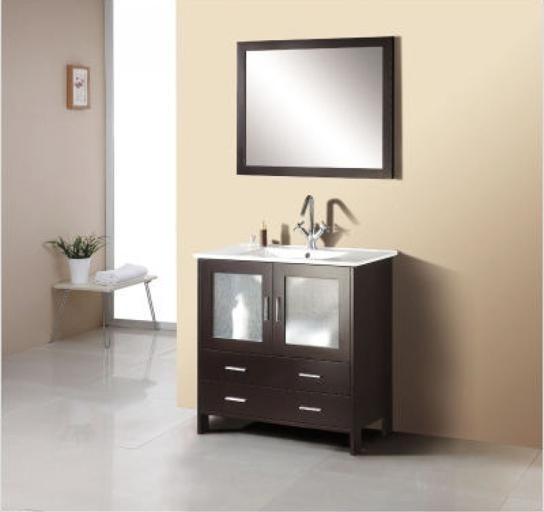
[67,64,90,110]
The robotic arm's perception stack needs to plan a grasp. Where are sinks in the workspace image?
[248,245,349,261]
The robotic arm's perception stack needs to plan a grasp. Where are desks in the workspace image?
[48,276,159,347]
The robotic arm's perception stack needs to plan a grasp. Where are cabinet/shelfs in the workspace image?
[197,257,393,455]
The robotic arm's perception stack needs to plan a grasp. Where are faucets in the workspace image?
[316,199,358,247]
[291,194,328,249]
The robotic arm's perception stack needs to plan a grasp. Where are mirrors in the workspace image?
[236,36,394,178]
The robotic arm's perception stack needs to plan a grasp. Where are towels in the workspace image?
[95,264,148,285]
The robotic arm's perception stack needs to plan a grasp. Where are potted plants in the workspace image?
[43,235,110,284]
